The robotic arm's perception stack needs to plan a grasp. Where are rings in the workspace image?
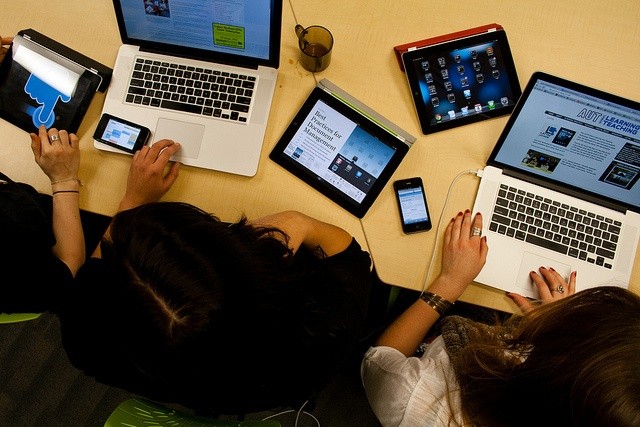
[470,226,482,238]
[49,134,60,143]
[550,284,565,294]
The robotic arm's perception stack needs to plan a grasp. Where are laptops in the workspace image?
[93,0,283,177]
[471,72,640,302]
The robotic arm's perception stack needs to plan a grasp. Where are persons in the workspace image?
[359,207,639,426]
[62,139,385,416]
[0,123,86,314]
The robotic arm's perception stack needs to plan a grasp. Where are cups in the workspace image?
[295,24,334,73]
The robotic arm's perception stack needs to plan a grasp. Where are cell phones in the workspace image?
[394,177,432,234]
[93,113,151,154]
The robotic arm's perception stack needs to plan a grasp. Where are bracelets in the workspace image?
[51,176,84,186]
[52,190,80,195]
[420,290,455,317]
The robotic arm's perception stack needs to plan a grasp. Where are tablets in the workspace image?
[268,86,411,218]
[400,27,521,136]
[0,33,104,144]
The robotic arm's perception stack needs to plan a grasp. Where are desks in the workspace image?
[0,0,636,320]
